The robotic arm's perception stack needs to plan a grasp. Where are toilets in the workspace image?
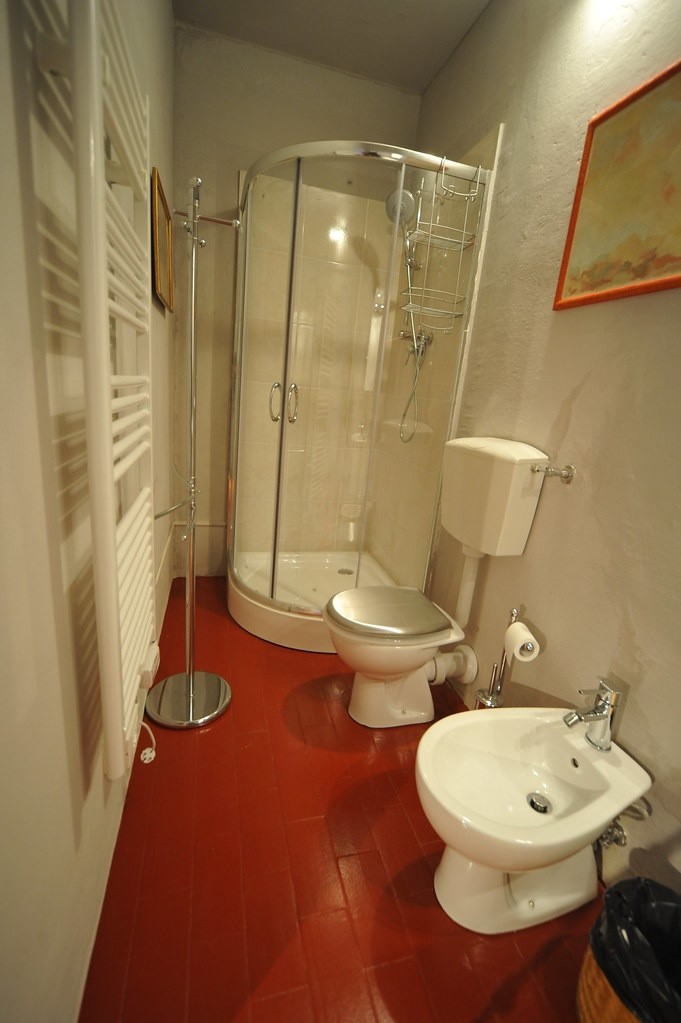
[322,436,550,729]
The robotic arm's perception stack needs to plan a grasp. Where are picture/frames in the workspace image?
[553,58,681,310]
[154,165,174,311]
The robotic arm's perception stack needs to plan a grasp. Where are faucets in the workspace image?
[562,679,621,750]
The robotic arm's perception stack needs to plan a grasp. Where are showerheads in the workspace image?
[385,188,416,267]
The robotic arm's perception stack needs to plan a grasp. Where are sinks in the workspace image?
[414,706,652,872]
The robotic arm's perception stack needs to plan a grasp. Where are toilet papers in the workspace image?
[502,622,541,667]
